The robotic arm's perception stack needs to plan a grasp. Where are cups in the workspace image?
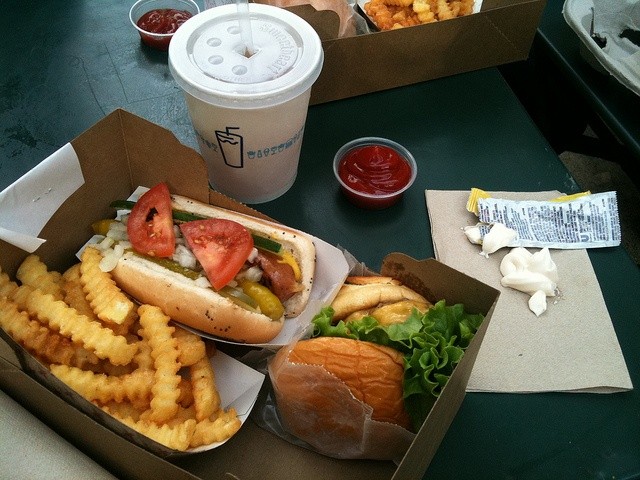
[168,7,324,206]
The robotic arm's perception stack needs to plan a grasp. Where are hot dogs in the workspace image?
[90,181,317,344]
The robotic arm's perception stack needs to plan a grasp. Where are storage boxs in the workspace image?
[210,1,546,104]
[2,110,500,480]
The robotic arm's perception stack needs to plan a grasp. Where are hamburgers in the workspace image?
[265,273,486,462]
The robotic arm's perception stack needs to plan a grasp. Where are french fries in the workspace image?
[0,245,242,452]
[363,1,480,32]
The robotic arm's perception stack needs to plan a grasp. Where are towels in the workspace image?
[425,189,635,394]
